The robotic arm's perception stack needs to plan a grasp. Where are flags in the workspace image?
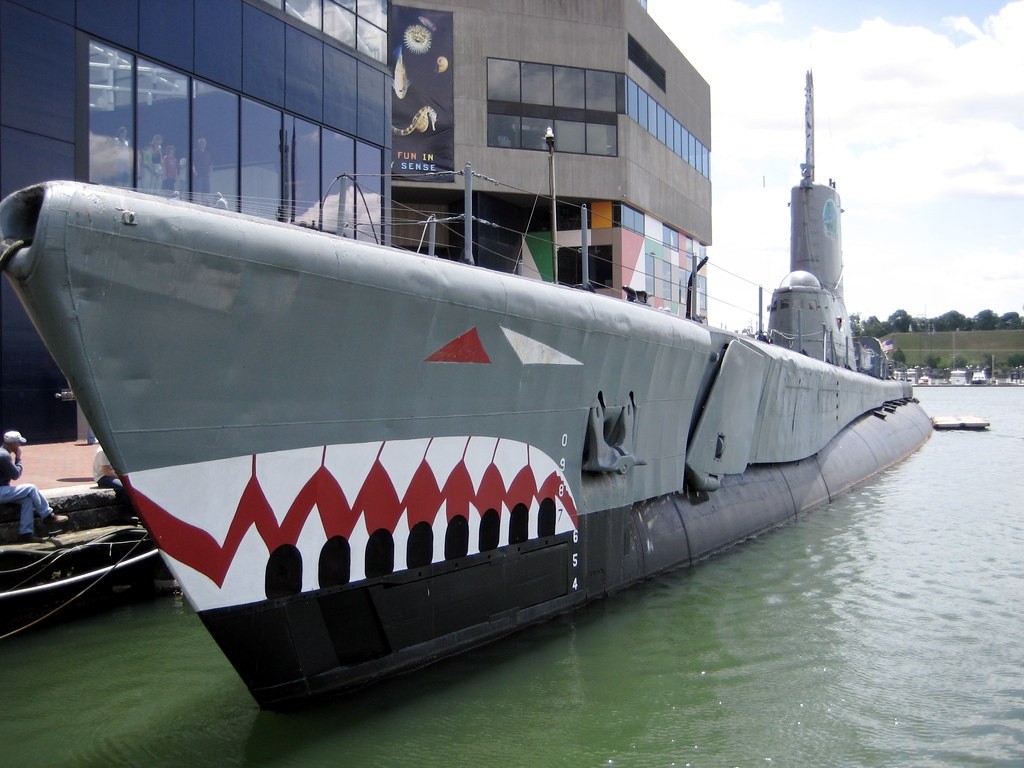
[881,338,894,351]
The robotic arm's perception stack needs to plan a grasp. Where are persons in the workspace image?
[0,431,69,542]
[116,126,214,205]
[92,445,140,523]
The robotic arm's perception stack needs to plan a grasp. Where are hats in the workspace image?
[4,430,26,443]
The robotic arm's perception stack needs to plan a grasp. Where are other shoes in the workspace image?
[43,513,69,524]
[17,533,43,543]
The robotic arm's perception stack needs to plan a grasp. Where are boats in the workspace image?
[0,67,932,713]
